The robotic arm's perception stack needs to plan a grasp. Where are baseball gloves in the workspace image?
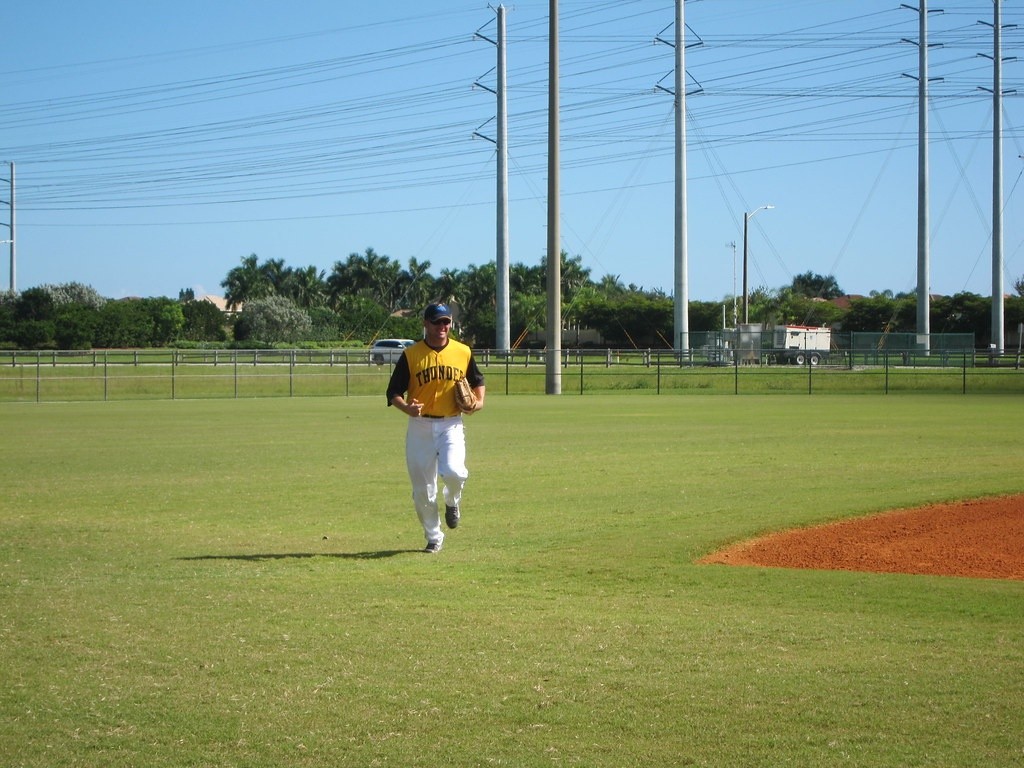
[453,378,477,412]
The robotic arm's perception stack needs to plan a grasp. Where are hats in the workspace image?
[424,304,452,321]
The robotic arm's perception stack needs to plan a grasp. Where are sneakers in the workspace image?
[445,504,460,529]
[425,542,443,552]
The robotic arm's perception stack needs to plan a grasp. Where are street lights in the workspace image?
[744,206,775,324]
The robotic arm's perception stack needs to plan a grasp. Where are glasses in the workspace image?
[429,320,450,325]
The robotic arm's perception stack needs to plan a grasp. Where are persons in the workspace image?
[387,303,485,554]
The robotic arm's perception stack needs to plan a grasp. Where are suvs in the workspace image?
[371,339,417,365]
